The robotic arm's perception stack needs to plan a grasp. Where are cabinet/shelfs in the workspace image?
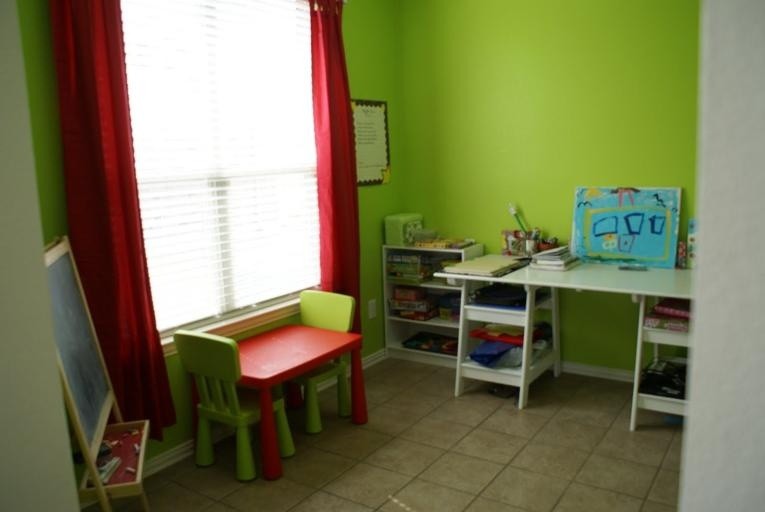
[379,240,485,368]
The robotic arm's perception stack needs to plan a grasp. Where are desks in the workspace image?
[186,323,368,482]
[432,253,691,435]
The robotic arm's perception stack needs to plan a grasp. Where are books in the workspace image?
[85,456,122,484]
[526,244,579,270]
[654,296,691,320]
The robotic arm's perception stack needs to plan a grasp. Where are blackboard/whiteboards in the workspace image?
[42,235,116,461]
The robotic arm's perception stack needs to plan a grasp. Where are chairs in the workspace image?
[167,288,358,484]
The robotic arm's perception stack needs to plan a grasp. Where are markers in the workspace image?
[618,265,649,271]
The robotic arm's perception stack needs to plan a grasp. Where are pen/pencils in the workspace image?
[508,201,529,234]
